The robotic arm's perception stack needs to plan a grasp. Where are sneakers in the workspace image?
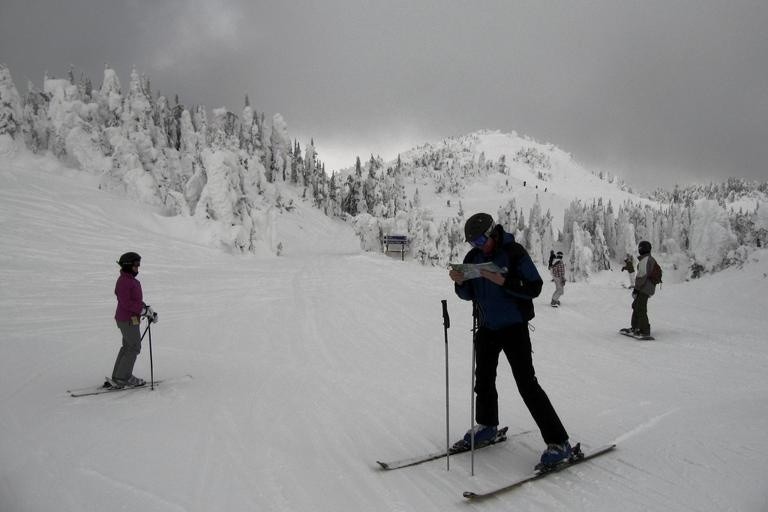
[112,376,147,388]
[463,424,498,445]
[551,300,558,305]
[624,325,650,337]
[541,440,573,465]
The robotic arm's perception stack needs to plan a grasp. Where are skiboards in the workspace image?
[67,380,162,396]
[376,426,616,499]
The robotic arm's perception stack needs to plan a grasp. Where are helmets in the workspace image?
[638,241,652,255]
[556,251,564,258]
[464,213,499,255]
[118,252,141,277]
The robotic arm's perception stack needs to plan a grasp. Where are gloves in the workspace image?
[561,278,566,286]
[631,289,639,299]
[140,306,158,324]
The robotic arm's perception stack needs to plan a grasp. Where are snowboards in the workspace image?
[619,329,654,340]
[552,304,558,308]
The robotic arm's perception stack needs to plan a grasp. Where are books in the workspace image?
[445,260,504,281]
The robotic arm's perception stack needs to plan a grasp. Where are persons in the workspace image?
[625,239,658,337]
[547,249,567,309]
[548,248,557,282]
[622,254,636,289]
[444,212,573,469]
[108,251,158,387]
